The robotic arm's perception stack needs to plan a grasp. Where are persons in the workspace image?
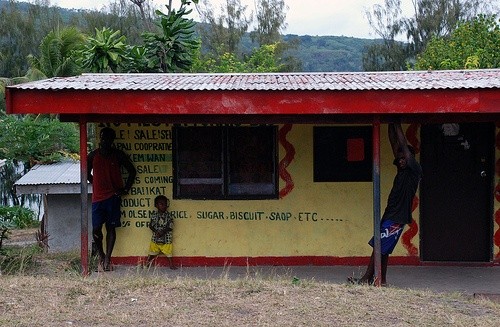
[348,122,421,285]
[143,194,178,270]
[87,127,138,271]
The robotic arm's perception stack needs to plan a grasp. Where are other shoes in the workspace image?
[348,276,386,286]
[98,257,110,270]
